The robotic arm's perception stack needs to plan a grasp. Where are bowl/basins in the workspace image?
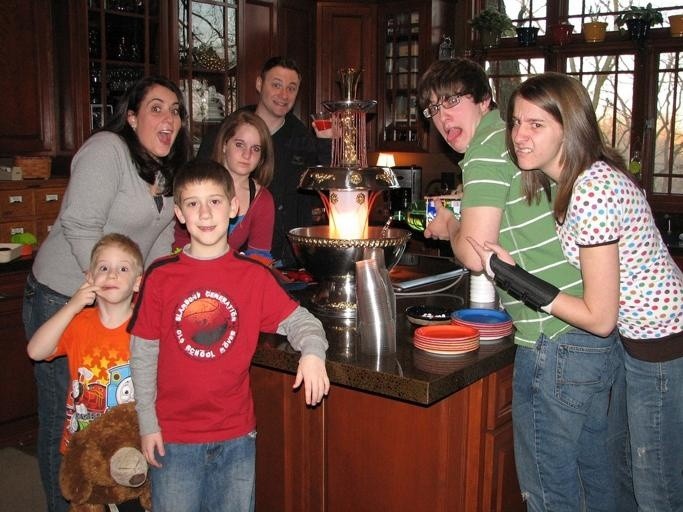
[470,276,497,304]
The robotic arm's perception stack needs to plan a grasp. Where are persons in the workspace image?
[172,108,276,270]
[197,53,331,268]
[25,231,145,454]
[465,69,683,512]
[417,54,638,512]
[20,72,195,512]
[127,157,333,512]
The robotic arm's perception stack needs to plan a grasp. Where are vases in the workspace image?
[517,22,608,45]
[668,14,683,38]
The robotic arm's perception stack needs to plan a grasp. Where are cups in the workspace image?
[311,112,333,131]
[355,247,398,356]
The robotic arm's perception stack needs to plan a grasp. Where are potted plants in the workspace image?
[613,3,663,39]
[466,6,514,49]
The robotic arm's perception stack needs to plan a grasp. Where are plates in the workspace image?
[405,306,515,357]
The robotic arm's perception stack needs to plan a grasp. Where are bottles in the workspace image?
[393,200,461,231]
[89,33,144,104]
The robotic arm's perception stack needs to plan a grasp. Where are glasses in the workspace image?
[421,92,469,119]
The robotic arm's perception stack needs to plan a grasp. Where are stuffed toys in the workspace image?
[58,400,152,512]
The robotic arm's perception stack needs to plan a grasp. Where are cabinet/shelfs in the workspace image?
[0,1,90,423]
[309,3,481,154]
[67,2,285,155]
